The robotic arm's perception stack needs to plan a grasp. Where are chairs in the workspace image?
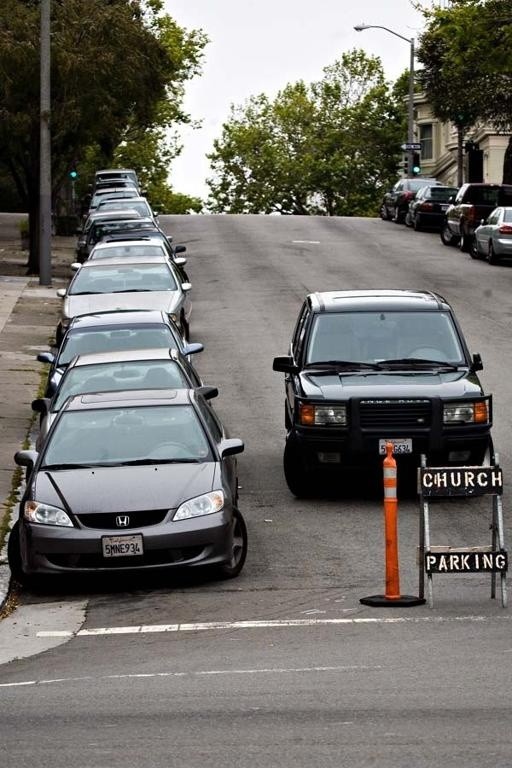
[319,314,442,363]
[142,365,173,387]
[83,330,169,354]
[86,375,112,390]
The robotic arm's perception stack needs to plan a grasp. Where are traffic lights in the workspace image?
[411,152,422,175]
[69,168,79,181]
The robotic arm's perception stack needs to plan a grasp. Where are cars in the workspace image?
[403,186,464,233]
[469,205,512,264]
[377,176,448,226]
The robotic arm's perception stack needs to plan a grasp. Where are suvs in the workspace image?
[272,285,498,506]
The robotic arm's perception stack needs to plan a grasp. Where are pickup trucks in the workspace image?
[440,181,512,254]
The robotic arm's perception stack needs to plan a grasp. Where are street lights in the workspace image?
[352,22,416,179]
